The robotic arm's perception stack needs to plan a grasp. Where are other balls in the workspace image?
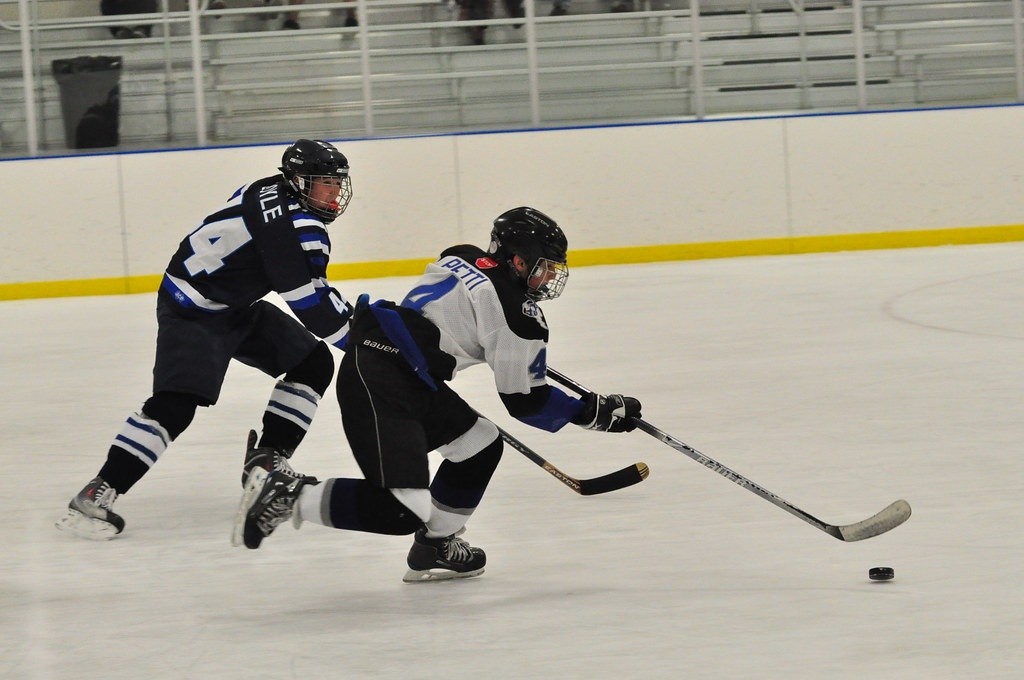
[868,567,894,580]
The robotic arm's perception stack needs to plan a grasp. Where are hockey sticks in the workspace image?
[327,285,652,499]
[543,363,915,545]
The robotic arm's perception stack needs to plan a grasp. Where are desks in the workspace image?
[0,0,1024,146]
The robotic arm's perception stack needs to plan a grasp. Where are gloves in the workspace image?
[580,392,642,434]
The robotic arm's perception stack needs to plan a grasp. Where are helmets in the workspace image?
[487,207,567,293]
[282,139,349,225]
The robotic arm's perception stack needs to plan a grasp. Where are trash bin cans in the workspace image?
[47,54,123,148]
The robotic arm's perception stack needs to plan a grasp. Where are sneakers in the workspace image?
[403,523,487,582]
[232,466,324,549]
[55,476,125,541]
[240,429,306,488]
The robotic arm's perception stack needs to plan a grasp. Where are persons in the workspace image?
[54,137,354,543]
[227,206,642,581]
[99,0,635,47]
